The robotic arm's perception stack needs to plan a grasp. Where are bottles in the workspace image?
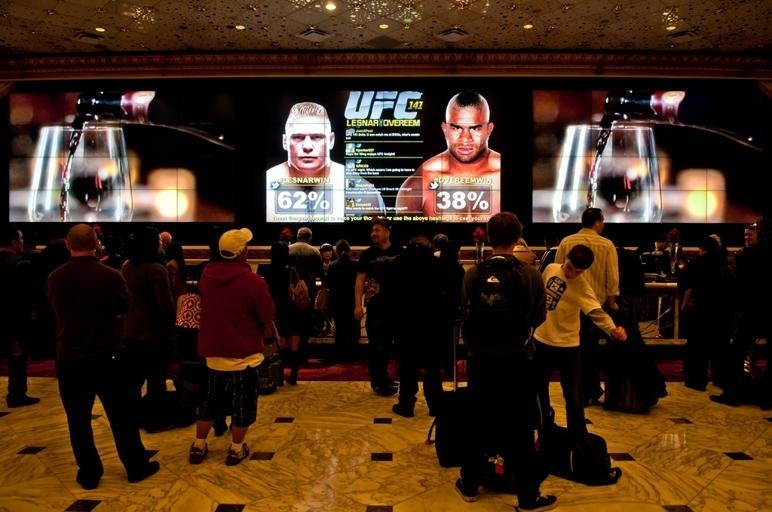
[601,86,768,154]
[71,88,233,151]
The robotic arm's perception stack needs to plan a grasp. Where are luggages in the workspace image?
[435,321,476,466]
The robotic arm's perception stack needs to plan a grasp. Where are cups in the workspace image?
[28,124,133,222]
[552,121,664,224]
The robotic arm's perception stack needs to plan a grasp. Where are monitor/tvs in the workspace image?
[0,77,251,245]
[521,77,772,245]
[251,77,521,245]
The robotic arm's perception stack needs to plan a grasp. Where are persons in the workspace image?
[261,223,469,419]
[0,221,41,409]
[710,219,770,406]
[394,91,503,222]
[454,211,559,512]
[666,230,736,393]
[46,224,277,490]
[265,98,387,223]
[530,208,627,432]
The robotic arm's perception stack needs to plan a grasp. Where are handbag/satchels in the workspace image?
[549,424,613,487]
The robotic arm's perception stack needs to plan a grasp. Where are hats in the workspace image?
[371,216,391,230]
[219,227,252,259]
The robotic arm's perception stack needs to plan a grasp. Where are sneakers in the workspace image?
[226,443,248,466]
[129,461,159,483]
[394,403,415,418]
[7,394,40,408]
[374,384,396,397]
[191,441,208,466]
[519,494,557,511]
[455,477,478,500]
